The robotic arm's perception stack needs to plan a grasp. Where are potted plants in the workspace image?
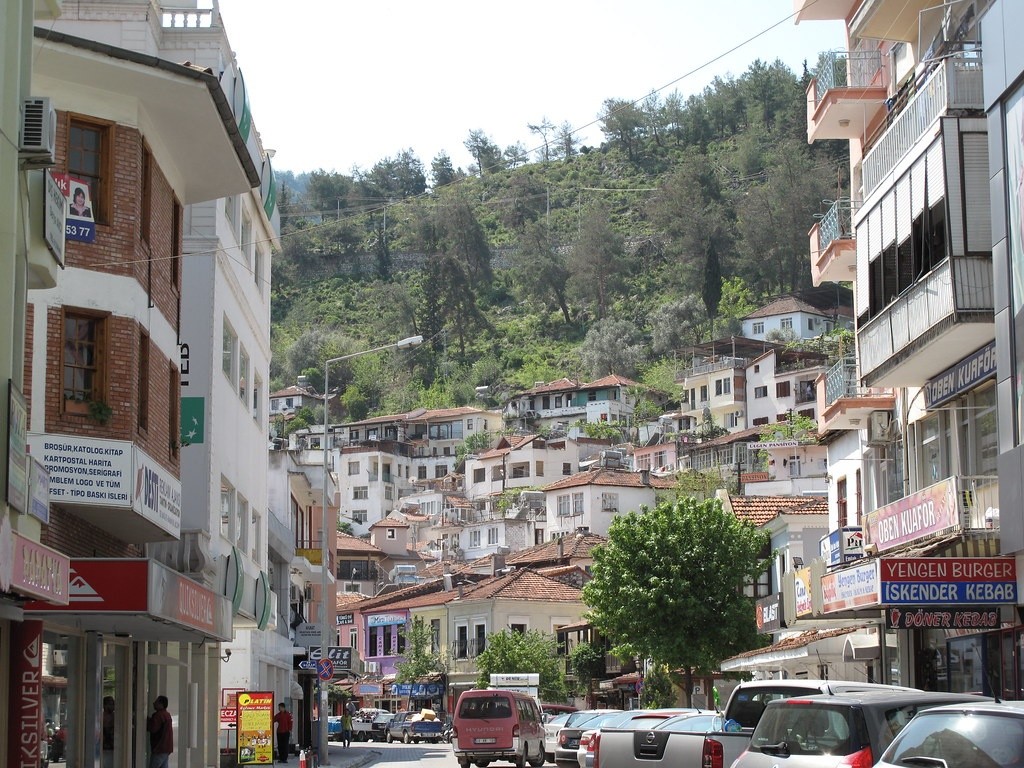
[65,397,113,424]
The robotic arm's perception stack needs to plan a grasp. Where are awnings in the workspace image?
[599,676,641,691]
[720,623,869,673]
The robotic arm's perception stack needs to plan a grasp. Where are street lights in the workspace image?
[320,335,425,764]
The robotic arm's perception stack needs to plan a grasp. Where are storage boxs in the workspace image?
[421,708,436,721]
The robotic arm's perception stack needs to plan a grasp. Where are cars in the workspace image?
[542,708,724,768]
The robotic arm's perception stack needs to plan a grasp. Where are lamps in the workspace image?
[791,557,803,571]
[864,542,878,559]
[848,419,861,425]
[840,119,850,127]
[848,265,857,273]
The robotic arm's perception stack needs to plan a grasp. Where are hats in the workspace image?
[348,698,352,701]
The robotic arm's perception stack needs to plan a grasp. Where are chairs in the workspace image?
[804,717,828,751]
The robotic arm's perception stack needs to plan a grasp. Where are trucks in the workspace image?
[329,706,389,742]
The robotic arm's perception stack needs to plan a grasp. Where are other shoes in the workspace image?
[278,759,288,763]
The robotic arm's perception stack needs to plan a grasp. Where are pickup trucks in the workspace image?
[385,711,442,744]
[585,679,925,768]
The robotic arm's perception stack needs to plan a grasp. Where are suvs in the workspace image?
[372,714,395,741]
[873,698,1024,768]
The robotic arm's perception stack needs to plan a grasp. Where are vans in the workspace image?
[730,692,1005,768]
[452,689,547,768]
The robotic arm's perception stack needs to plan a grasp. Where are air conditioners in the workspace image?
[374,660,380,673]
[734,410,743,418]
[815,317,822,324]
[867,411,889,444]
[364,661,368,672]
[19,95,57,164]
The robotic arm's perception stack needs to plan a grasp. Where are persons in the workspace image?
[146,696,173,768]
[102,696,115,750]
[341,709,353,748]
[274,703,294,764]
[70,188,92,218]
[346,698,356,717]
[45,719,54,744]
